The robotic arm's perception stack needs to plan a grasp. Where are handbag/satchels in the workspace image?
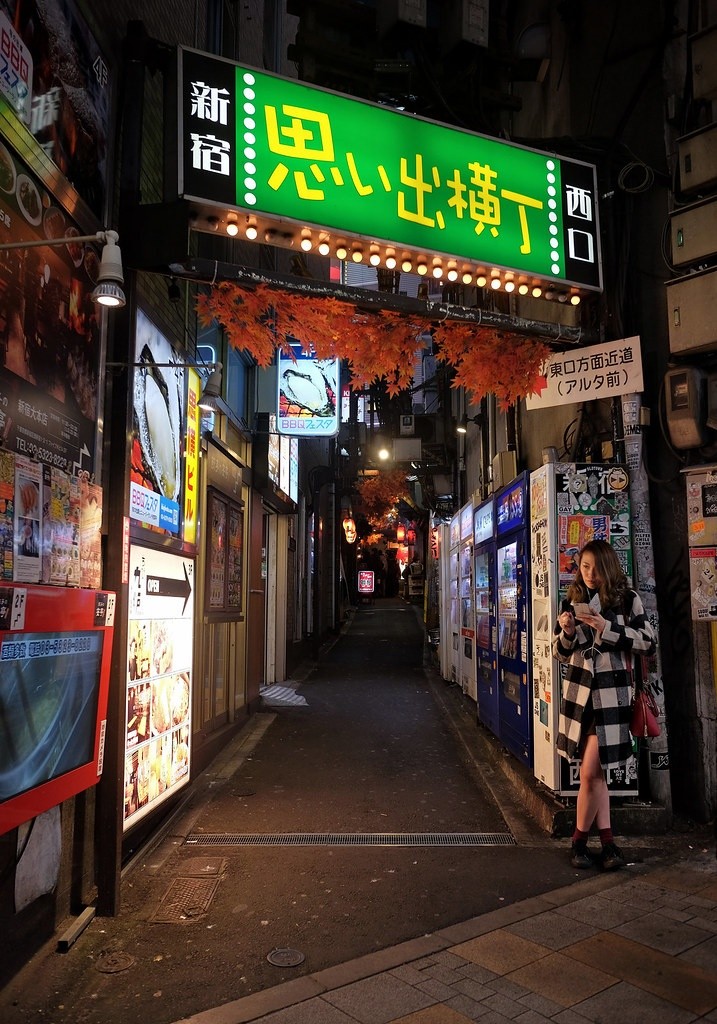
[630,690,662,739]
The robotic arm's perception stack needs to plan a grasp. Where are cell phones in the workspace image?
[574,602,591,616]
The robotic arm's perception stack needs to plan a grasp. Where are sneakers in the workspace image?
[602,844,625,870]
[571,841,591,867]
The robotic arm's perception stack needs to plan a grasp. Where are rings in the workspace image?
[563,616,566,620]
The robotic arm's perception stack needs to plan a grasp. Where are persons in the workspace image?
[549,539,657,870]
